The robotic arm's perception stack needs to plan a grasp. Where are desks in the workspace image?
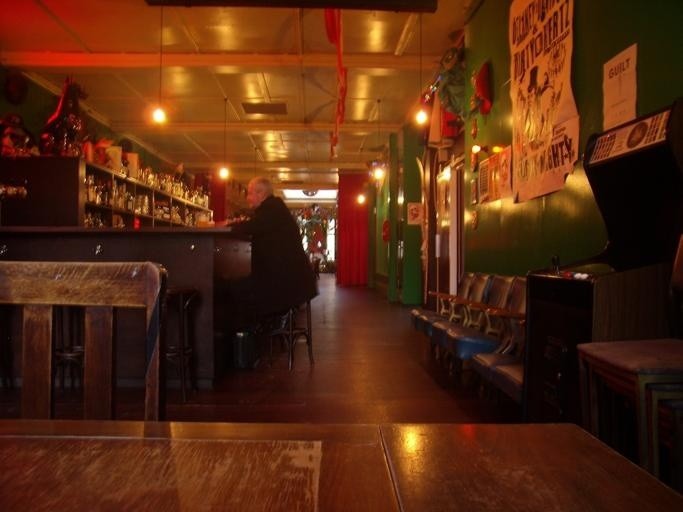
[0,418,683,512]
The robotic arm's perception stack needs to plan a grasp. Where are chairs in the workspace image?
[0,261,169,421]
[409,271,526,408]
[575,233,682,477]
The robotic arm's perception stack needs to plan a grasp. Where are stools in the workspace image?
[248,295,312,373]
[159,285,199,403]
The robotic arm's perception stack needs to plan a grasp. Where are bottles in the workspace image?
[84,166,213,228]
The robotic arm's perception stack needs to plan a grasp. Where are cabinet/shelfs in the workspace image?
[0,153,214,228]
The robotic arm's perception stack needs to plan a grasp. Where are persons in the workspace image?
[187,176,320,387]
